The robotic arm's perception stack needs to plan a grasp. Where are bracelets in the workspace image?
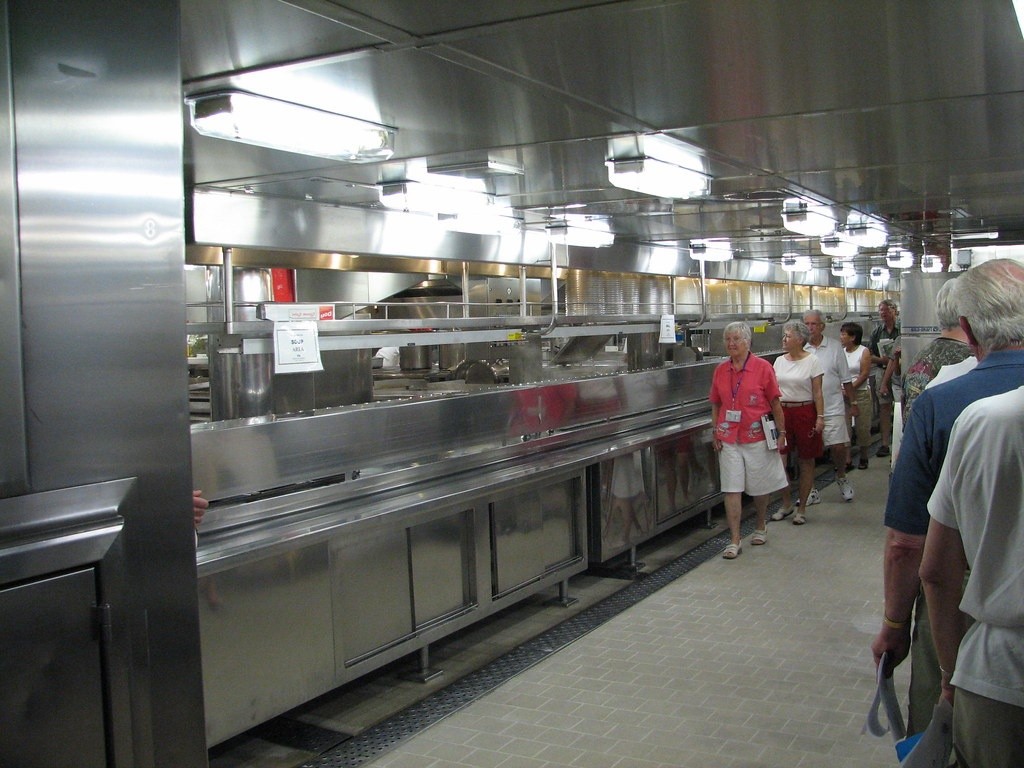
[883,612,909,628]
[817,415,823,417]
[779,431,786,434]
[939,666,953,675]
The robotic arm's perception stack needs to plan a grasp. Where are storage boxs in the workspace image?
[761,416,788,450]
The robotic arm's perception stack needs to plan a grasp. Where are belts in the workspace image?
[781,401,812,407]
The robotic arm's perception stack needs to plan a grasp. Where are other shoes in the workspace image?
[876,446,890,457]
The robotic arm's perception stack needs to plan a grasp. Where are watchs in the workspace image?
[850,400,857,405]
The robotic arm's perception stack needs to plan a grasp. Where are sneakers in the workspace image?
[796,489,820,506]
[836,475,854,500]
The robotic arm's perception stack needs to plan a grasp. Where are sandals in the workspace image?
[772,505,793,520]
[858,458,868,469]
[834,461,855,472]
[793,513,807,525]
[723,540,742,558]
[751,525,767,544]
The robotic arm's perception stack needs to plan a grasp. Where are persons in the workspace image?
[873,258,1024,768]
[868,300,901,457]
[794,310,858,506]
[833,323,873,471]
[773,321,826,525]
[708,322,785,558]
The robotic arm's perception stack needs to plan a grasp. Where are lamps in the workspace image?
[604,156,715,202]
[780,197,840,221]
[780,211,840,237]
[846,226,890,249]
[921,264,943,273]
[820,241,859,257]
[830,267,856,277]
[689,247,734,263]
[377,178,492,216]
[781,252,812,262]
[951,231,1000,240]
[870,273,890,281]
[780,261,813,272]
[546,226,617,249]
[182,89,400,168]
[437,212,521,238]
[886,256,914,269]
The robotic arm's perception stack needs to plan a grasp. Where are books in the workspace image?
[761,415,788,450]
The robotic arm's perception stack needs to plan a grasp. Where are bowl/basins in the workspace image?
[372,357,384,368]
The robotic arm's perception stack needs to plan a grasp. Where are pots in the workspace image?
[465,357,509,384]
[399,343,431,370]
[440,344,465,369]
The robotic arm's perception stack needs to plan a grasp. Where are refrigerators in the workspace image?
[899,271,970,379]
